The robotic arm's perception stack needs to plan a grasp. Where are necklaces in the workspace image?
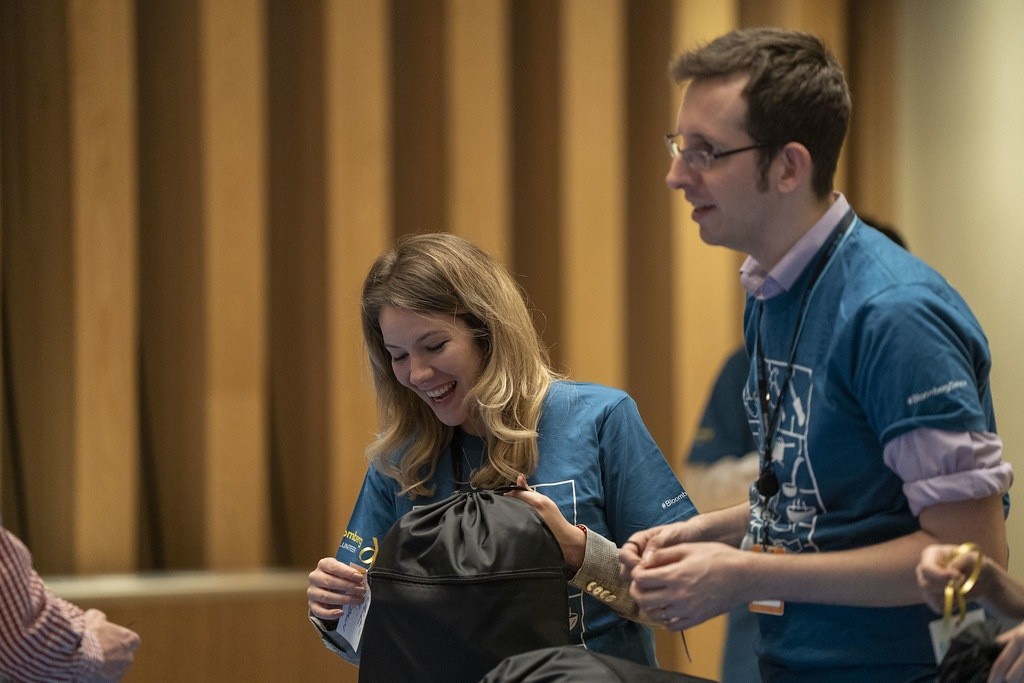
[460,442,485,491]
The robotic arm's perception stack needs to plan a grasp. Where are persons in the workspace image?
[306,231,701,683]
[914,541,1024,683]
[0,528,140,683]
[683,217,911,683]
[619,27,1014,683]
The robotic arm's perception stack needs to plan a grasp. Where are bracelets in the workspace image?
[578,526,587,534]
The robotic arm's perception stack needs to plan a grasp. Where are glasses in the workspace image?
[664,131,782,171]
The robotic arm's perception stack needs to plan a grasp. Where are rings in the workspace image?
[627,541,640,555]
[661,609,679,624]
